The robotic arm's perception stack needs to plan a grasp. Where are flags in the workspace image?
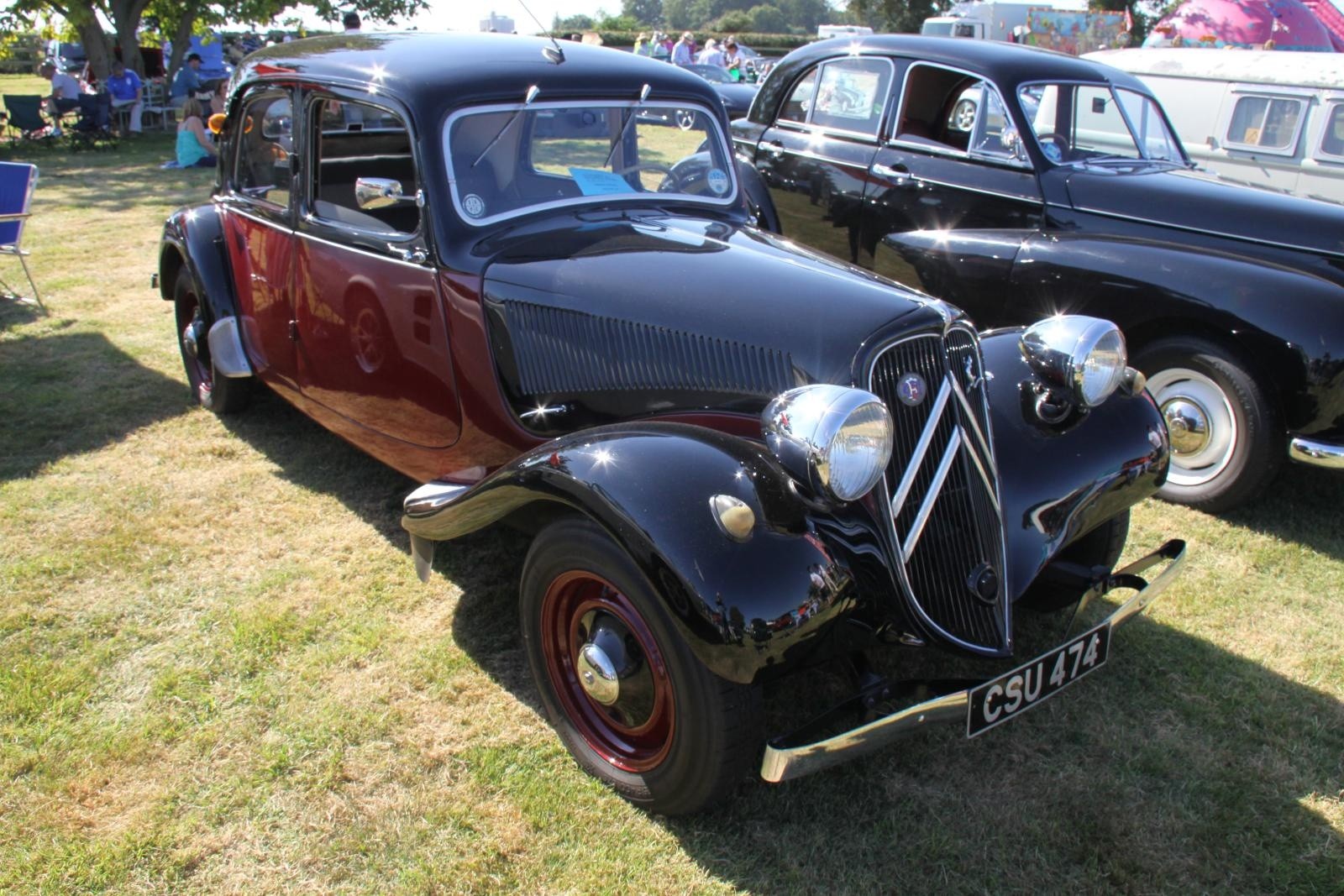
[1125,8,1133,31]
[1272,16,1290,33]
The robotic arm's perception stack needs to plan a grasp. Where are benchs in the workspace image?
[296,155,494,216]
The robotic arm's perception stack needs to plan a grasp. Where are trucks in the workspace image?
[920,1,1130,57]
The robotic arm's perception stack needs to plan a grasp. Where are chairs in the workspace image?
[117,83,152,132]
[63,93,113,150]
[2,94,51,155]
[0,160,45,316]
[150,85,181,130]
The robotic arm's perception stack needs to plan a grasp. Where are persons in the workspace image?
[719,35,746,82]
[108,60,145,138]
[38,62,83,136]
[228,31,263,66]
[689,42,697,60]
[169,54,211,111]
[82,61,96,84]
[698,38,725,79]
[175,97,218,169]
[671,31,694,66]
[204,78,229,128]
[635,32,674,56]
[329,12,381,128]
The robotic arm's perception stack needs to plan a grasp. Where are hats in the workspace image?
[637,32,649,40]
[683,31,693,45]
[188,53,205,63]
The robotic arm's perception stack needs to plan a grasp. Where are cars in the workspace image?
[727,34,1343,513]
[151,31,1187,820]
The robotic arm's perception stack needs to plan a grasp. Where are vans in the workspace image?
[1032,48,1343,206]
[817,24,873,42]
